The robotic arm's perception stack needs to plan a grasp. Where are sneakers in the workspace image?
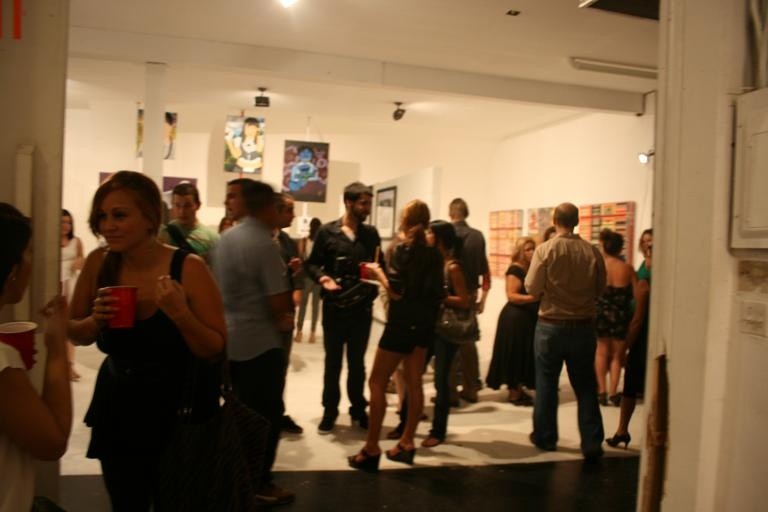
[279,415,303,434]
[318,390,478,469]
[509,391,630,461]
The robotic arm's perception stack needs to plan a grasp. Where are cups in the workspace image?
[0,321,39,371]
[359,261,370,280]
[106,285,140,329]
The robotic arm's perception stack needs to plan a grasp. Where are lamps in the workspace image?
[570,56,658,81]
[255,87,270,107]
[635,151,655,163]
[393,100,405,121]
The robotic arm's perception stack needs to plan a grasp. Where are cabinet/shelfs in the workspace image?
[727,84,767,261]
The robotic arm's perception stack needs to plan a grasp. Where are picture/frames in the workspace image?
[373,185,397,241]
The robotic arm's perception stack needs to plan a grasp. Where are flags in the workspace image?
[281,139,330,203]
[135,109,181,161]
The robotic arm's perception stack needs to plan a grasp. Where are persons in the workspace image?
[286,147,322,191]
[159,185,221,273]
[209,178,303,500]
[347,199,491,472]
[61,209,84,382]
[161,112,175,160]
[525,203,604,455]
[306,183,386,433]
[593,227,654,450]
[295,217,323,343]
[63,169,228,511]
[485,227,556,404]
[1,199,73,511]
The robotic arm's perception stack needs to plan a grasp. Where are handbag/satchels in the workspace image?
[435,303,479,343]
